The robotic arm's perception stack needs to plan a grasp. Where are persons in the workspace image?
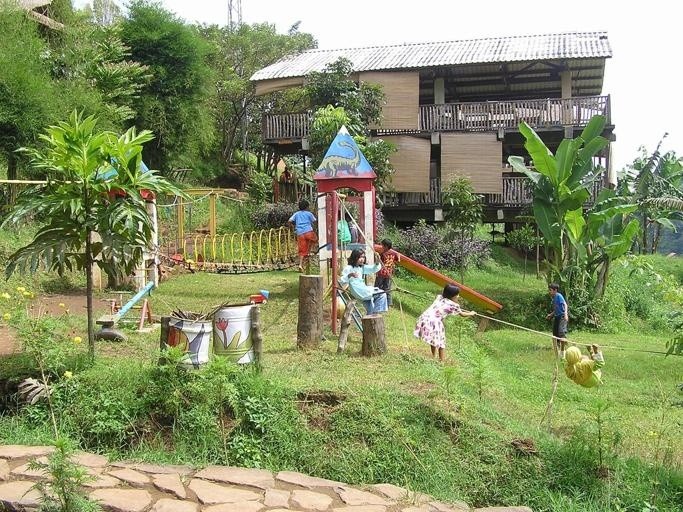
[286,200,321,274]
[561,342,605,389]
[343,212,362,243]
[541,285,571,357]
[410,284,477,359]
[334,209,351,247]
[339,246,389,314]
[373,238,400,309]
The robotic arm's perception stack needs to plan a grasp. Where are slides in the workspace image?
[373,243,503,314]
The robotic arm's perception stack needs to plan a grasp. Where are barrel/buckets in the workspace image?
[168,311,213,369]
[160,316,171,367]
[212,302,255,364]
